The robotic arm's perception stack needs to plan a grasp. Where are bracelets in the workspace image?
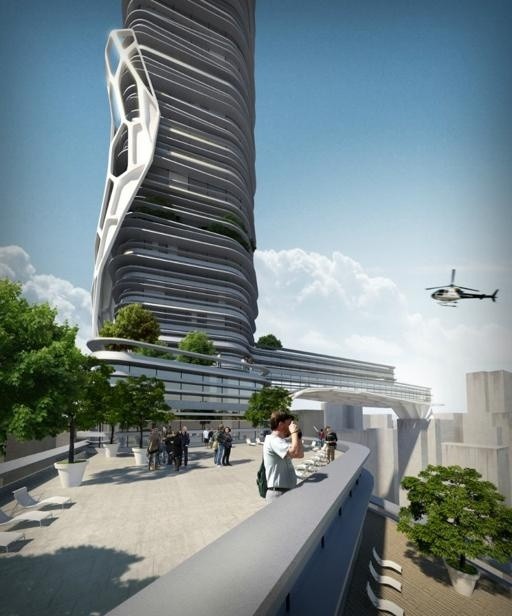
[291,431,298,434]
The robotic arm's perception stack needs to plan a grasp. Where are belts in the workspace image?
[268,488,289,491]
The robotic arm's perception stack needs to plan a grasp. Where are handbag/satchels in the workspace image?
[257,460,267,497]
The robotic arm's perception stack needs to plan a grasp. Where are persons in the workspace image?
[325,426,337,464]
[319,429,325,447]
[263,411,304,505]
[147,424,232,472]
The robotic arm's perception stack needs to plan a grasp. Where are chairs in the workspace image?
[294,440,327,480]
[245,437,264,447]
[365,547,405,616]
[0,485,73,553]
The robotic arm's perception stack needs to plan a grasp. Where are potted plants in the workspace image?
[6,323,176,489]
[394,462,511,597]
